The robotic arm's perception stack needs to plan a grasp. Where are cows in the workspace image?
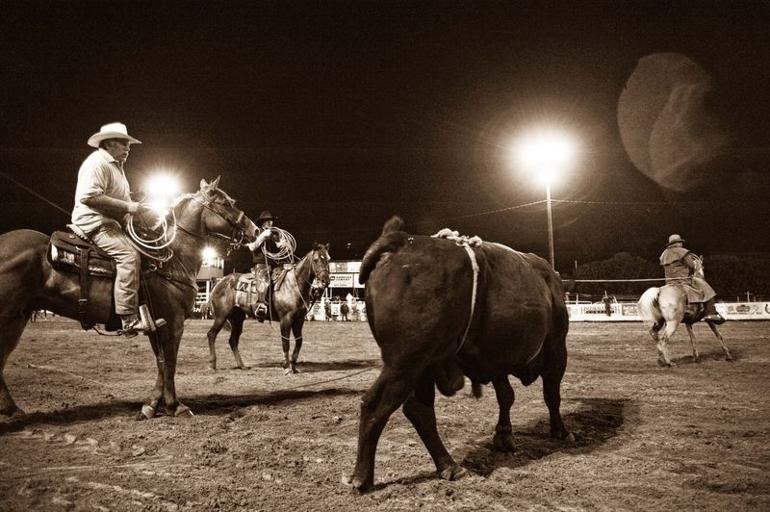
[346,215,575,496]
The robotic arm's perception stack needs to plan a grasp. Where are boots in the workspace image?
[118,313,168,338]
[257,302,265,322]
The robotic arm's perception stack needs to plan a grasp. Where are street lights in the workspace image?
[508,129,579,271]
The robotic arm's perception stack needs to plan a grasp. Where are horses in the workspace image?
[636,255,733,370]
[206,242,332,376]
[0,174,261,422]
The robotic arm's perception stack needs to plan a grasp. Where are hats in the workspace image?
[666,234,685,245]
[254,211,278,225]
[87,121,143,148]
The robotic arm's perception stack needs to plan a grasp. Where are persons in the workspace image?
[200,300,208,320]
[341,302,350,321]
[602,293,614,316]
[248,206,285,320]
[69,121,165,340]
[658,233,728,325]
